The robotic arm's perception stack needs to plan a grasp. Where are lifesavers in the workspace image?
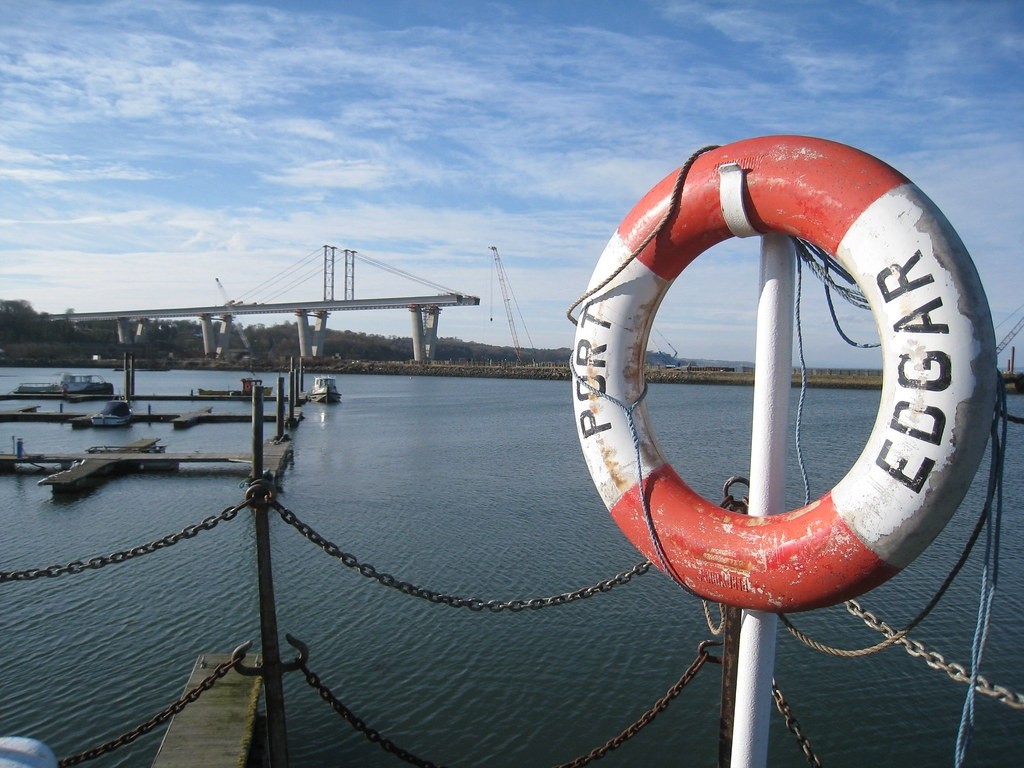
[567,134,997,612]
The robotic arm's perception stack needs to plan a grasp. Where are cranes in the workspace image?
[488,246,536,366]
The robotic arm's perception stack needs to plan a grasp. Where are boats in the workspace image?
[91,394,133,426]
[309,376,342,403]
[13,373,114,395]
[198,378,274,396]
